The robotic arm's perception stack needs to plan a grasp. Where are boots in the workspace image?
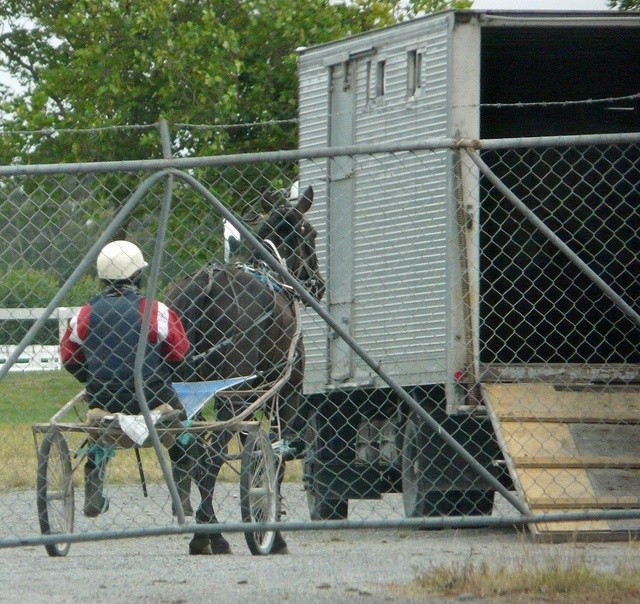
[171,465,193,516]
[84,463,109,516]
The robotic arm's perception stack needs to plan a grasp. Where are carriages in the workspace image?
[31,185,325,556]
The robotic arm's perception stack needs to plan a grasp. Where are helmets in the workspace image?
[97,240,148,280]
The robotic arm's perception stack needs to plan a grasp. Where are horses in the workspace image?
[162,184,325,557]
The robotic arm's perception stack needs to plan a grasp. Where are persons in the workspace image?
[61,239,197,517]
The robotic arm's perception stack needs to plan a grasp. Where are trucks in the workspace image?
[295,10,639,539]
